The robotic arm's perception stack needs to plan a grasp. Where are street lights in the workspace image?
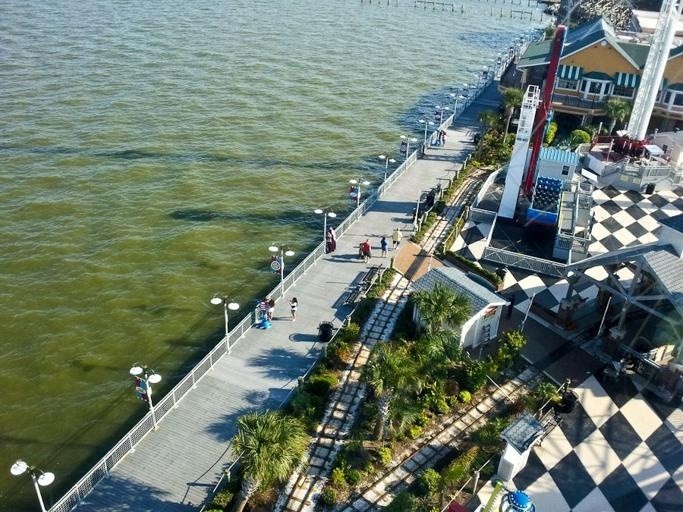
[210,297,239,354]
[434,28,535,124]
[418,120,434,153]
[10,461,55,512]
[268,244,294,299]
[378,155,395,193]
[400,135,416,169]
[130,362,161,431]
[313,209,336,256]
[349,181,369,222]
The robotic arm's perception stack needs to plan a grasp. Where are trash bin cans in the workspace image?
[319,320,334,342]
[428,193,435,206]
[473,132,481,144]
[645,183,655,194]
[562,391,576,413]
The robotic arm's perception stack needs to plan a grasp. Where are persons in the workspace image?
[260,297,266,320]
[589,135,598,152]
[433,128,442,147]
[266,299,275,320]
[442,131,446,148]
[326,230,336,253]
[362,239,371,263]
[356,243,364,260]
[329,227,335,241]
[380,237,387,257]
[392,228,402,250]
[288,297,297,321]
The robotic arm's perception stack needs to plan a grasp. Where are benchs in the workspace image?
[343,285,365,305]
[358,262,383,284]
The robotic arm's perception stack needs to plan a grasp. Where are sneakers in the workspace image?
[291,317,297,322]
